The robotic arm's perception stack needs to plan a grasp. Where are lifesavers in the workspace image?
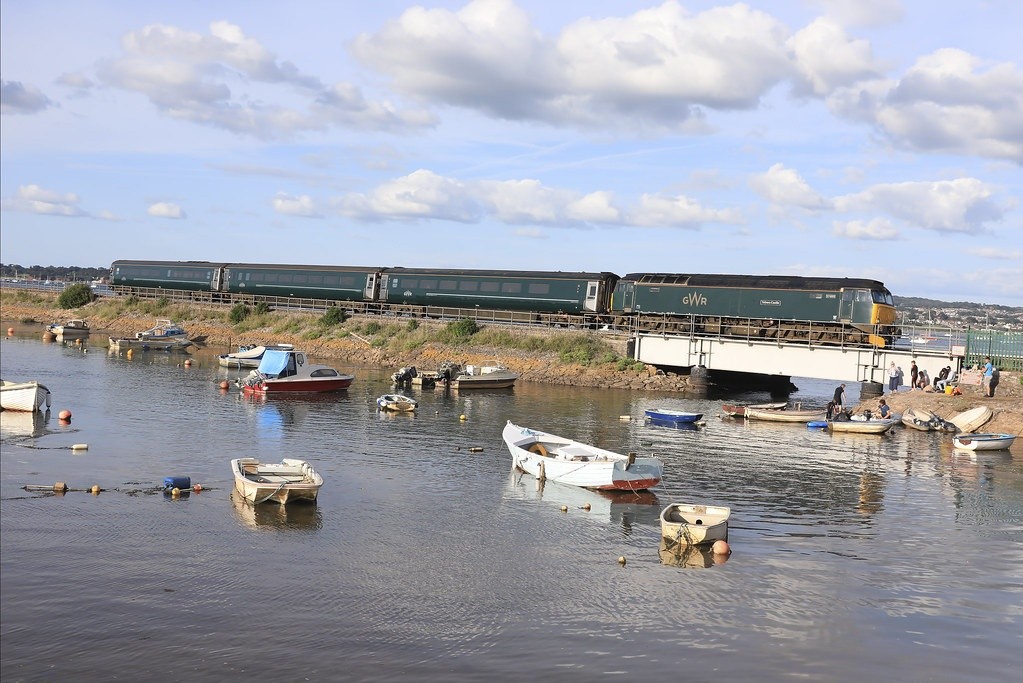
[528,443,548,457]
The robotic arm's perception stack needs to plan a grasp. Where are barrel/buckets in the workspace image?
[945,386,953,394]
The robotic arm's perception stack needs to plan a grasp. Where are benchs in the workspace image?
[948,374,961,387]
[244,471,306,481]
[670,511,726,525]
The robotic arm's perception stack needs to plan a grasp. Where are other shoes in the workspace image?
[937,389,945,393]
[983,394,991,398]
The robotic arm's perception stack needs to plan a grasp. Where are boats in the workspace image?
[0,379,50,412]
[721,400,789,418]
[658,535,730,570]
[0,409,45,438]
[233,349,356,395]
[948,405,994,434]
[661,502,732,545]
[389,358,520,390]
[214,343,297,368]
[45,318,90,335]
[826,418,899,435]
[900,405,956,433]
[502,418,665,490]
[644,407,703,423]
[501,458,660,524]
[106,317,193,352]
[230,485,320,535]
[230,456,325,507]
[377,393,418,412]
[742,404,828,424]
[644,418,701,432]
[951,432,1017,452]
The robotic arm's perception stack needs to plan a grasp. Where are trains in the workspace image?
[106,257,905,353]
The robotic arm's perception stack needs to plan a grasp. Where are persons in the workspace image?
[916,371,927,390]
[878,400,890,419]
[934,366,955,390]
[833,384,847,414]
[887,362,900,393]
[910,360,918,391]
[982,357,993,397]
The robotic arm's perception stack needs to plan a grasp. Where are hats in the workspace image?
[983,356,990,360]
